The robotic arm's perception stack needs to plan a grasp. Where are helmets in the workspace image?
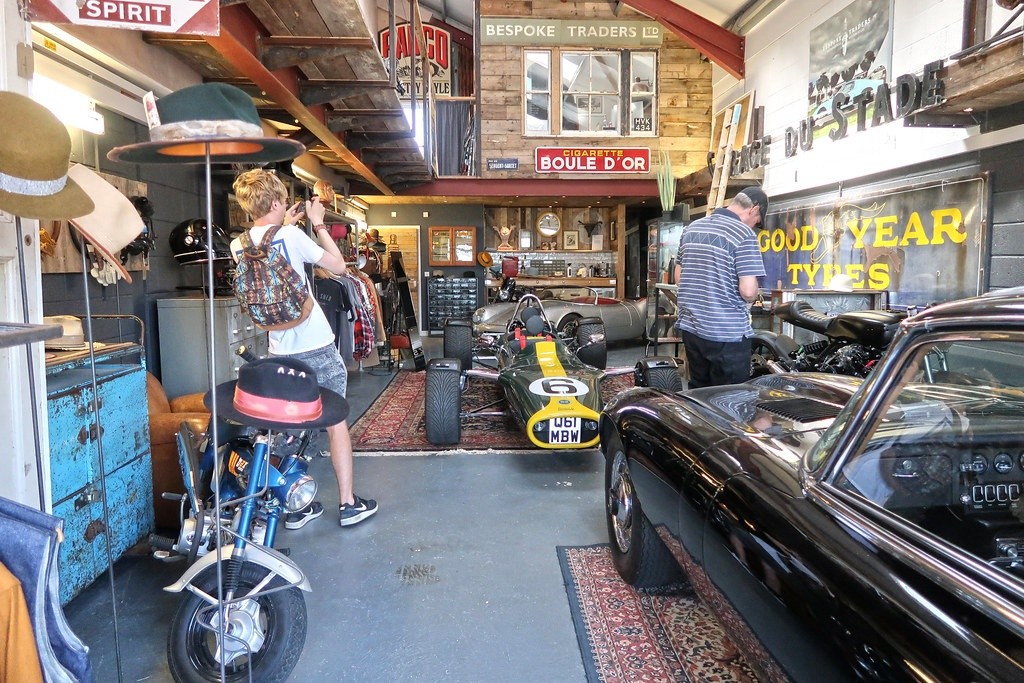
[169,218,233,266]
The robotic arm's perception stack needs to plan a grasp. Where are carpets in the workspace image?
[555,544,762,683]
[317,365,635,457]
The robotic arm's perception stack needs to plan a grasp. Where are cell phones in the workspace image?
[296,202,312,213]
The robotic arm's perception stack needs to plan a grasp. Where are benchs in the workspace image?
[571,297,619,304]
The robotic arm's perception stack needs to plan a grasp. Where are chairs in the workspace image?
[525,316,545,337]
[521,307,538,323]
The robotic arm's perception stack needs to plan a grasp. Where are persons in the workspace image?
[673,187,769,391]
[231,168,379,530]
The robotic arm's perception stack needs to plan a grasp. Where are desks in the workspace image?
[655,282,678,356]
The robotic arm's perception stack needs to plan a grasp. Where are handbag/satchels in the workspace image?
[390,334,411,349]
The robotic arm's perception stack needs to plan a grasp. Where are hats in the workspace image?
[741,187,769,230]
[202,357,350,430]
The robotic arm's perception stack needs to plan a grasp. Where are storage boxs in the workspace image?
[535,288,616,301]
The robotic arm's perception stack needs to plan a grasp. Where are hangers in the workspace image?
[314,265,359,279]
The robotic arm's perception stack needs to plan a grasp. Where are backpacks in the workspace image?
[232,224,314,332]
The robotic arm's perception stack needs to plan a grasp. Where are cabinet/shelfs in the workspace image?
[427,277,478,336]
[157,294,269,402]
[428,225,476,267]
[646,217,693,344]
[483,203,625,307]
[45,314,157,609]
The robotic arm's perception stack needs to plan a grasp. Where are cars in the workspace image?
[596,284,1024,683]
[471,292,677,347]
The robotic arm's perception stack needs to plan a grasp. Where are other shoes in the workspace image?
[339,493,379,527]
[284,501,324,530]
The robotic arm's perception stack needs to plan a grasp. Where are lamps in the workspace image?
[631,77,654,102]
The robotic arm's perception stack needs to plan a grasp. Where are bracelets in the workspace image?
[314,224,327,232]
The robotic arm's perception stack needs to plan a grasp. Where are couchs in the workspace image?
[147,370,212,528]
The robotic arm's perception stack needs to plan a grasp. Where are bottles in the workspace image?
[567,264,572,277]
[668,258,675,284]
[588,266,592,277]
[521,261,525,274]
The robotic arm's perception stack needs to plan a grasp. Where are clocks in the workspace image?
[536,211,561,238]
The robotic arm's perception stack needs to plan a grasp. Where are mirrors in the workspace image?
[391,251,426,371]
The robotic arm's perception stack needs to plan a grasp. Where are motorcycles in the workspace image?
[486,268,553,305]
[749,301,951,385]
[149,348,329,683]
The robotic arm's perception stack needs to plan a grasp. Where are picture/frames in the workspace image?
[562,230,579,250]
[224,190,251,239]
[709,89,756,171]
[576,96,605,116]
[752,169,995,312]
